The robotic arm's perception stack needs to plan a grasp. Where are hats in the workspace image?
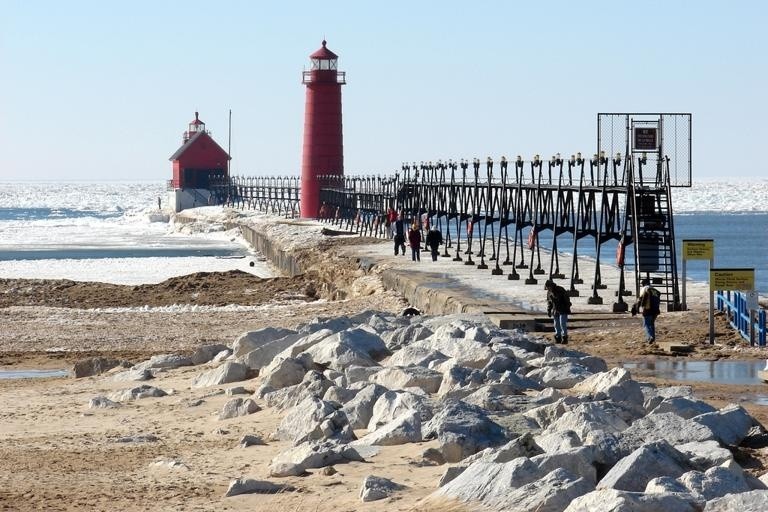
[412,224,416,228]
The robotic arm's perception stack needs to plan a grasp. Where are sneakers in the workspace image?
[647,337,654,344]
[560,339,567,344]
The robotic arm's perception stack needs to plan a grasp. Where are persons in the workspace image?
[157,196,162,210]
[383,203,443,262]
[544,280,572,346]
[635,278,661,344]
